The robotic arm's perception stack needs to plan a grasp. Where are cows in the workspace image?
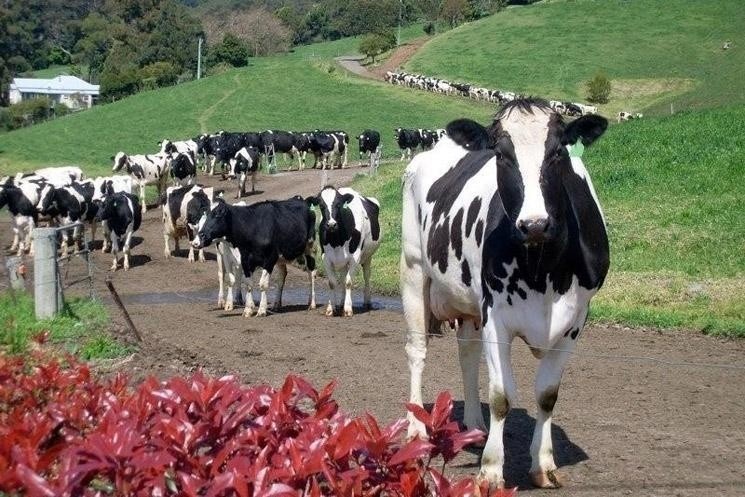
[311,186,383,318]
[384,71,526,107]
[198,195,320,318]
[394,93,611,489]
[0,166,142,273]
[157,128,383,199]
[108,151,174,205]
[160,183,211,263]
[615,111,644,124]
